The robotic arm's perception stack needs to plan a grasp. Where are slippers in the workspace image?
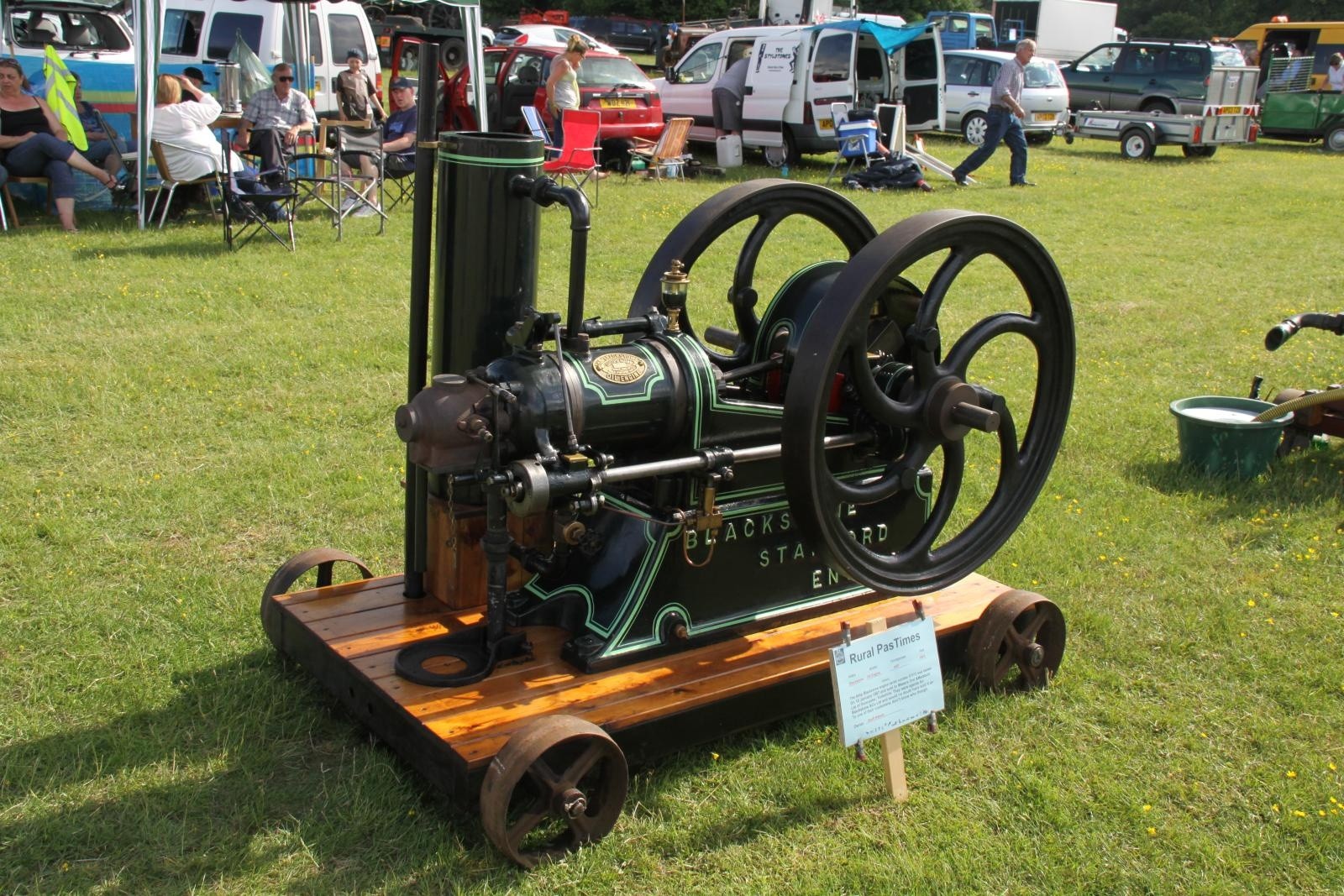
[99,171,117,189]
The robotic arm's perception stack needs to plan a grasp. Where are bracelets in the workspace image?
[1014,104,1018,107]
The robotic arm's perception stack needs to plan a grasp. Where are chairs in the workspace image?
[624,117,695,186]
[505,66,540,116]
[541,108,601,209]
[521,104,567,178]
[18,17,56,43]
[0,101,416,253]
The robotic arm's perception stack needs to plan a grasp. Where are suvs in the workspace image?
[1049,34,1261,163]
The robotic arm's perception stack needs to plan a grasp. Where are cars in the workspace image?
[386,36,666,162]
[366,0,752,118]
[944,48,1071,148]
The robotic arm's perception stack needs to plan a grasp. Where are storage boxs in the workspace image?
[838,119,878,156]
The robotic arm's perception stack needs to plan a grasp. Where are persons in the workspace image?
[148,63,318,224]
[546,34,611,183]
[952,39,1037,187]
[1245,48,1344,103]
[711,56,751,139]
[336,48,418,218]
[0,57,138,235]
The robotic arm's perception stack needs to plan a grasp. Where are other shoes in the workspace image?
[952,170,969,187]
[591,171,611,181]
[1011,180,1035,187]
[269,170,283,190]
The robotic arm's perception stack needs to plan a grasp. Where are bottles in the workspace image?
[783,164,788,177]
[667,165,676,178]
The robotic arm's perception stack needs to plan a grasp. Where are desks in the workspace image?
[207,106,255,148]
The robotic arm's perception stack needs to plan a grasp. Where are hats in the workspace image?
[184,66,212,85]
[346,48,365,62]
[392,76,413,89]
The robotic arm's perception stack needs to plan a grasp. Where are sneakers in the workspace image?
[340,198,364,211]
[350,203,382,218]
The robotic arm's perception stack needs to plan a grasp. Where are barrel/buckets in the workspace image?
[1169,396,1295,480]
[215,60,242,113]
[716,135,742,168]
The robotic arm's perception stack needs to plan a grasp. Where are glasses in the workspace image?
[274,76,295,82]
[580,53,585,60]
[0,57,19,65]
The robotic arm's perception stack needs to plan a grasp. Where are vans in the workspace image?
[1206,14,1344,154]
[0,0,382,195]
[650,17,947,171]
